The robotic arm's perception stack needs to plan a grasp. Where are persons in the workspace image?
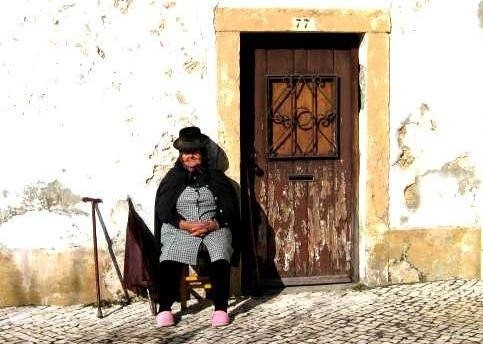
[156,126,237,327]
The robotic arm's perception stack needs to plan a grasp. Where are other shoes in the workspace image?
[211,310,230,329]
[156,310,174,327]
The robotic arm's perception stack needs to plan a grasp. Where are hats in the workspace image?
[173,126,210,152]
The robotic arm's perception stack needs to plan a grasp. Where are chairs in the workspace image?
[177,259,212,314]
